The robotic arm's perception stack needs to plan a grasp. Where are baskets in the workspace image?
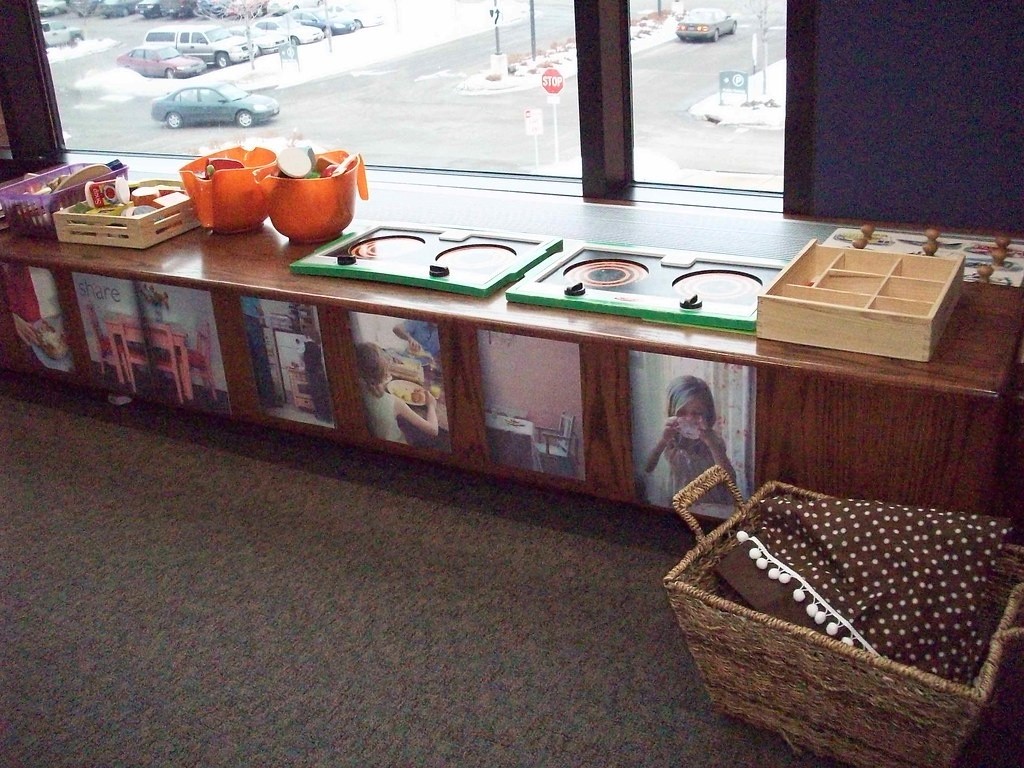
[660,467,1024,768]
[0,163,128,238]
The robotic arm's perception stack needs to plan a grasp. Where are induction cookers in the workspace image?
[507,242,789,333]
[289,223,563,298]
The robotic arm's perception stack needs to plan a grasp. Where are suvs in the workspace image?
[144,23,259,69]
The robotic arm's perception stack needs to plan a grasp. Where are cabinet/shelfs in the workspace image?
[274,328,318,412]
[0,181,1024,530]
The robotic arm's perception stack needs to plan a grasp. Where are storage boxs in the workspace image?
[51,179,201,250]
[757,239,967,361]
[0,162,132,238]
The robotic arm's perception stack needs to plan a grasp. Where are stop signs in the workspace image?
[541,68,565,94]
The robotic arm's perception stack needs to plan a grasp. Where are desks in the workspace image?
[103,310,188,399]
[389,358,450,451]
[485,413,543,473]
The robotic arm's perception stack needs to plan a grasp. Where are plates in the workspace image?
[388,380,435,406]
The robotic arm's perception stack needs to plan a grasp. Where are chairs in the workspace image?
[535,411,575,473]
[188,320,218,400]
[87,304,131,382]
[117,314,185,406]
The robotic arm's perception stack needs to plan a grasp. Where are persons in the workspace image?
[3,263,60,346]
[393,320,440,360]
[645,375,736,502]
[356,342,439,444]
[240,295,330,422]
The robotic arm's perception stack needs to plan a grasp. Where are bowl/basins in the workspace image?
[180,147,277,235]
[252,149,369,242]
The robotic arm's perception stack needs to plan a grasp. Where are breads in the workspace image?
[33,320,69,361]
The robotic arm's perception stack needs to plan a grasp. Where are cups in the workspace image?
[85,177,130,209]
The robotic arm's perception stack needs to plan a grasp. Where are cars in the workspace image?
[114,44,207,79]
[267,16,325,44]
[40,18,86,51]
[281,7,357,36]
[226,23,289,55]
[36,0,68,19]
[66,0,387,29]
[675,7,738,43]
[151,80,280,129]
